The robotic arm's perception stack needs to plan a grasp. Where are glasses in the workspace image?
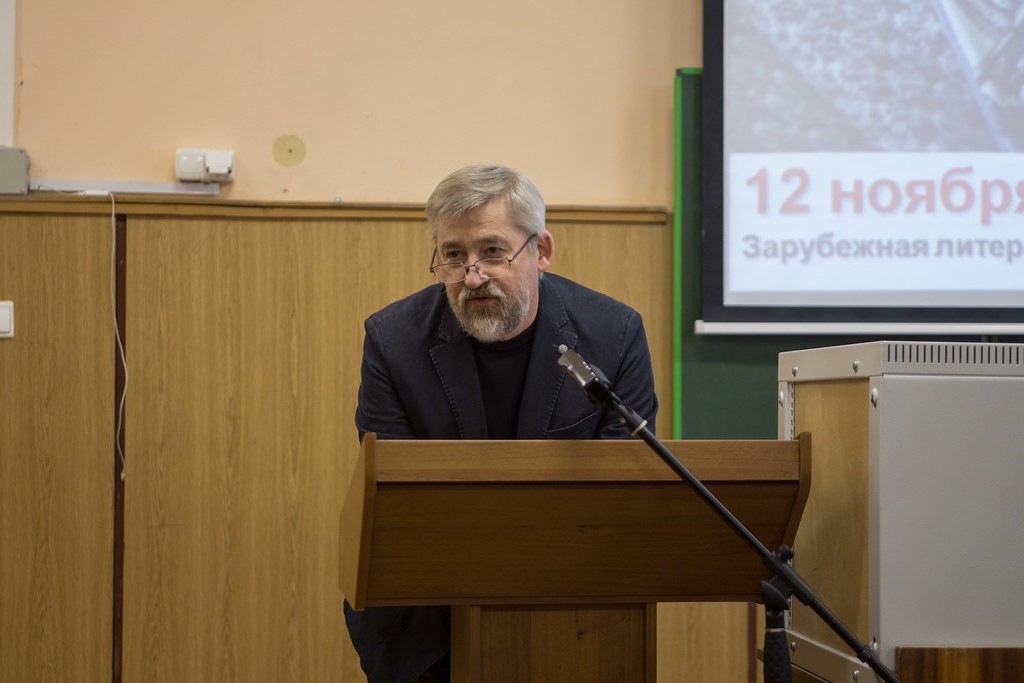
[428,233,537,283]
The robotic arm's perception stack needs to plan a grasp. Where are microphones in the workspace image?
[559,349,607,401]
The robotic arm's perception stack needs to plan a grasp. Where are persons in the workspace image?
[343,165,658,683]
